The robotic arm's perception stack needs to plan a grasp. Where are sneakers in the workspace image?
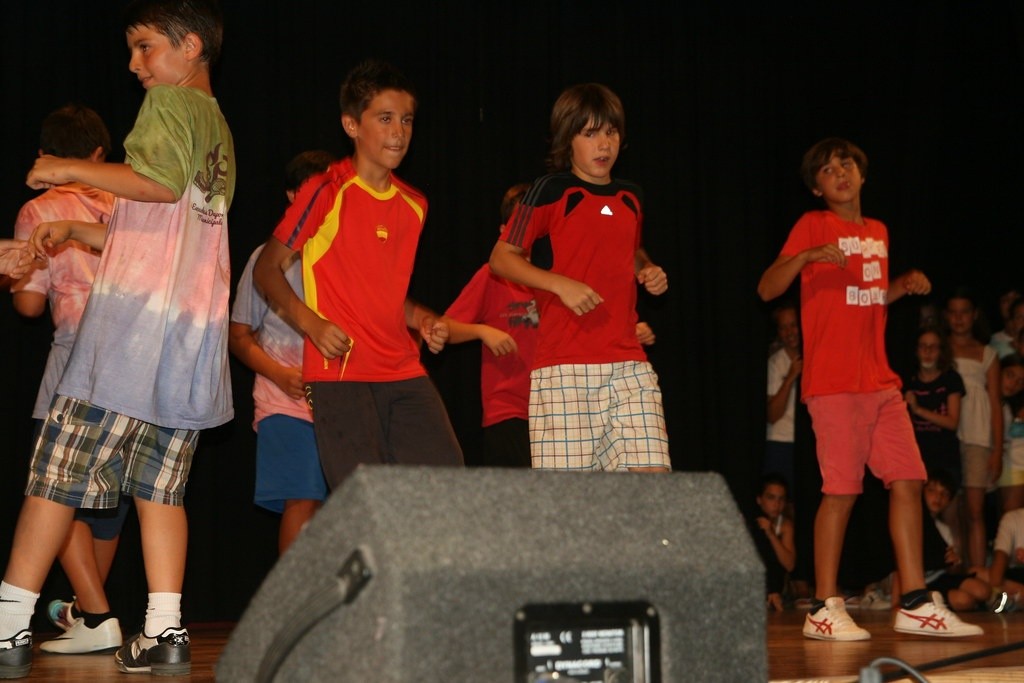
[115,624,191,678]
[802,596,870,640]
[39,617,123,656]
[46,599,78,631]
[0,628,32,679]
[895,590,984,637]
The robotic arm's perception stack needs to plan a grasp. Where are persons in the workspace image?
[995,354,1024,432]
[753,478,800,612]
[757,138,985,641]
[989,290,1022,358]
[1008,299,1024,353]
[890,478,997,614]
[0,0,237,677]
[0,239,36,280]
[11,106,125,657]
[942,290,1002,566]
[487,82,668,470]
[228,149,336,555]
[254,57,464,491]
[766,306,803,489]
[437,182,657,470]
[990,508,1024,612]
[901,327,967,565]
